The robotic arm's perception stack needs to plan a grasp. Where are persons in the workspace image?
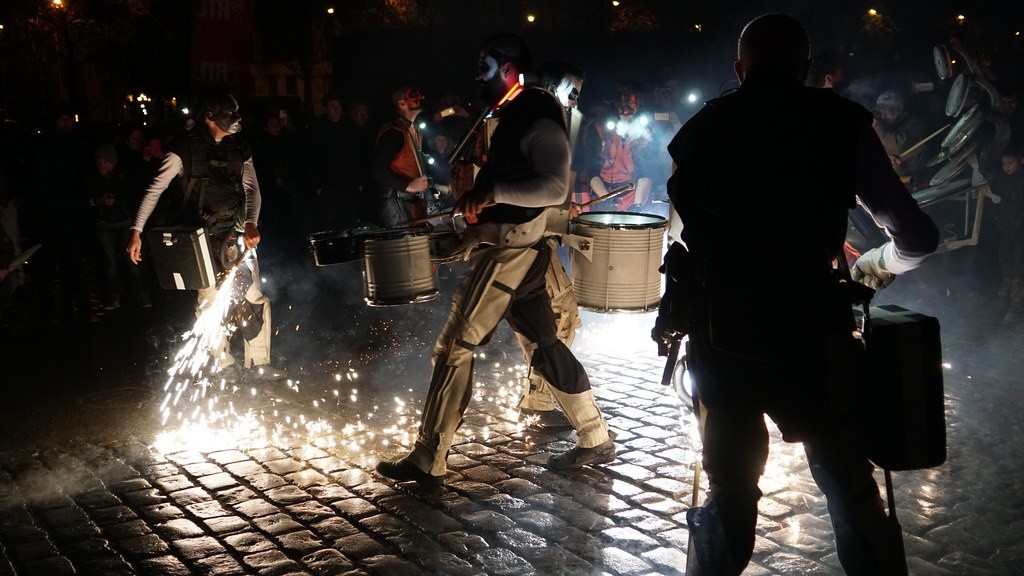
[124,93,289,381]
[804,53,1024,325]
[652,80,684,131]
[581,79,657,212]
[371,85,429,228]
[516,61,583,424]
[425,91,479,206]
[666,13,941,576]
[376,35,617,485]
[249,92,370,252]
[87,125,166,310]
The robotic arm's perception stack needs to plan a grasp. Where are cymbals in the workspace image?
[926,151,947,168]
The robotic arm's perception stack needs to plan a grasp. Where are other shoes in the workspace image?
[991,295,1023,323]
[136,293,152,308]
[104,295,120,310]
[547,436,616,469]
[243,363,287,385]
[376,457,444,486]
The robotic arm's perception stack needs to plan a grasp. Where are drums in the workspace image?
[933,43,963,80]
[426,228,465,264]
[909,177,977,254]
[944,72,989,125]
[942,102,994,164]
[929,159,973,187]
[358,222,440,309]
[567,210,669,314]
[309,229,366,266]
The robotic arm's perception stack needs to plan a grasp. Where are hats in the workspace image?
[94,144,117,166]
